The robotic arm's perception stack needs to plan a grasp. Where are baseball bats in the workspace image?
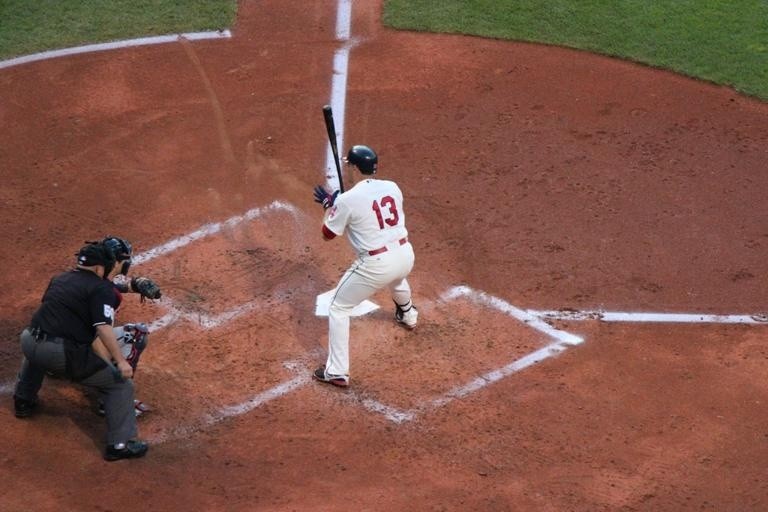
[322,104,344,195]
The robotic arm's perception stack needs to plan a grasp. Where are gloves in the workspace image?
[314,186,339,210]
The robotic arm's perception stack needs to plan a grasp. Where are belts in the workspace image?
[26,326,57,343]
[369,239,406,256]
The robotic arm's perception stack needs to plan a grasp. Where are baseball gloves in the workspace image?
[132,276,161,299]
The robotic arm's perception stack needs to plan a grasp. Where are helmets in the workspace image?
[78,235,133,278]
[341,145,378,175]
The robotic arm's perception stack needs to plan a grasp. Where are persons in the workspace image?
[312,144,419,386]
[94,235,163,416]
[12,241,149,462]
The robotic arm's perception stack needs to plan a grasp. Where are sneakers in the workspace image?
[15,395,38,418]
[314,368,349,385]
[105,442,148,461]
[98,399,145,416]
[394,314,416,329]
